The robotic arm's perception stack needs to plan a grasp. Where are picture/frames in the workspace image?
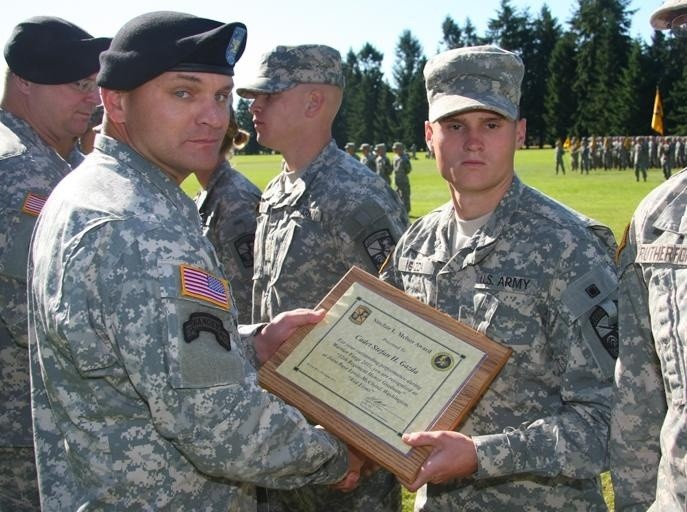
[254,263,517,485]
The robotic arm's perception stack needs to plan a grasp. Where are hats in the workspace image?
[374,143,387,152]
[359,143,371,151]
[423,45,524,122]
[95,12,247,91]
[344,142,356,150]
[236,44,345,100]
[88,106,104,128]
[553,136,686,144]
[3,16,112,85]
[649,1,686,31]
[392,142,405,151]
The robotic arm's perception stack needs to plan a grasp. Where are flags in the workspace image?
[650,88,664,136]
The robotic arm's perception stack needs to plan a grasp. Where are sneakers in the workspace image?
[554,163,686,182]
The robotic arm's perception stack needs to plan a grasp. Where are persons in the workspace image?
[79,105,105,155]
[555,134,687,182]
[609,0,687,512]
[236,43,411,512]
[0,16,113,512]
[343,140,417,214]
[26,12,369,512]
[190,103,263,325]
[337,46,619,512]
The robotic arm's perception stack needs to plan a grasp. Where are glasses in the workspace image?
[70,80,100,93]
[665,14,686,40]
